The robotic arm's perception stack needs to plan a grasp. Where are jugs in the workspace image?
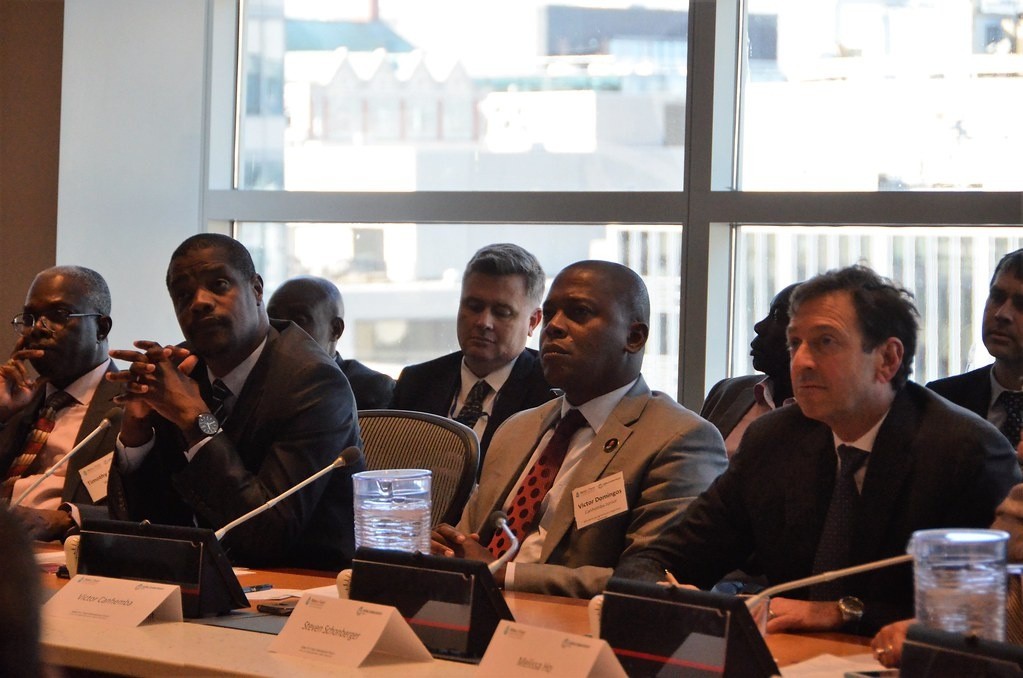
[352,469,433,558]
[906,527,1011,643]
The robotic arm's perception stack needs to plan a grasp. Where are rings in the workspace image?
[768,609,775,618]
[876,648,884,654]
[137,374,141,382]
[8,358,14,365]
[885,645,893,653]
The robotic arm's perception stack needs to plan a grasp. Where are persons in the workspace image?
[105,233,366,572]
[430,261,731,599]
[612,263,1023,633]
[389,243,567,487]
[262,274,397,412]
[870,482,1023,669]
[0,264,127,546]
[924,248,1023,451]
[698,280,806,464]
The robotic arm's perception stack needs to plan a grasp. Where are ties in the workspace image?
[0,390,74,506]
[997,392,1023,448]
[210,378,234,434]
[455,380,491,429]
[488,409,587,562]
[807,444,871,602]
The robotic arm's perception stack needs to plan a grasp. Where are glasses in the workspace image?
[10,309,103,337]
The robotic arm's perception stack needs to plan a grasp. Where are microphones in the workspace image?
[9,407,125,512]
[487,512,518,576]
[213,446,362,541]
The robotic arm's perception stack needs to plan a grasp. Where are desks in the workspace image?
[37,545,893,677]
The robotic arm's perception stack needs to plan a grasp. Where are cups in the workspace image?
[733,595,771,637]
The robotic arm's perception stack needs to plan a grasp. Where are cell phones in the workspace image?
[257,603,297,615]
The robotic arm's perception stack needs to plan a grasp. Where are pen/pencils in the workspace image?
[664,569,679,586]
[241,584,274,593]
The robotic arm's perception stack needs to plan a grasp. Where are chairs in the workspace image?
[358,410,482,532]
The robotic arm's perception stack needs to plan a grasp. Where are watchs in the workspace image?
[837,595,863,633]
[183,412,220,442]
[59,505,80,545]
